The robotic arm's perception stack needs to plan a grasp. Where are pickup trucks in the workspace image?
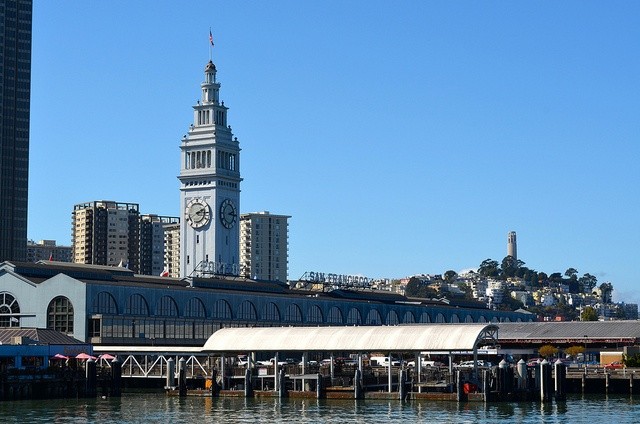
[407,358,434,367]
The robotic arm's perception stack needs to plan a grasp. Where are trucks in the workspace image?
[370,357,400,366]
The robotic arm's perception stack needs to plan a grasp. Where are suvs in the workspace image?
[454,360,487,368]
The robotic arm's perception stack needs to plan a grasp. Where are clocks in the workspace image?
[184,199,211,230]
[220,199,238,230]
